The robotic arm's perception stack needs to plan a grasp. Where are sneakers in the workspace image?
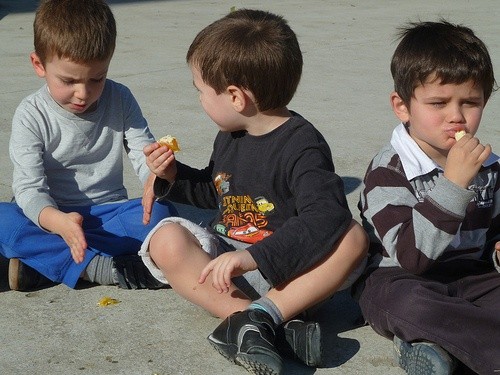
[9,258,49,290]
[280,315,324,367]
[207,308,283,375]
[111,252,166,290]
[391,333,459,375]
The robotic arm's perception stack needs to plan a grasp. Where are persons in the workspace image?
[357,15,500,375]
[142,6,371,374]
[0,1,176,293]
